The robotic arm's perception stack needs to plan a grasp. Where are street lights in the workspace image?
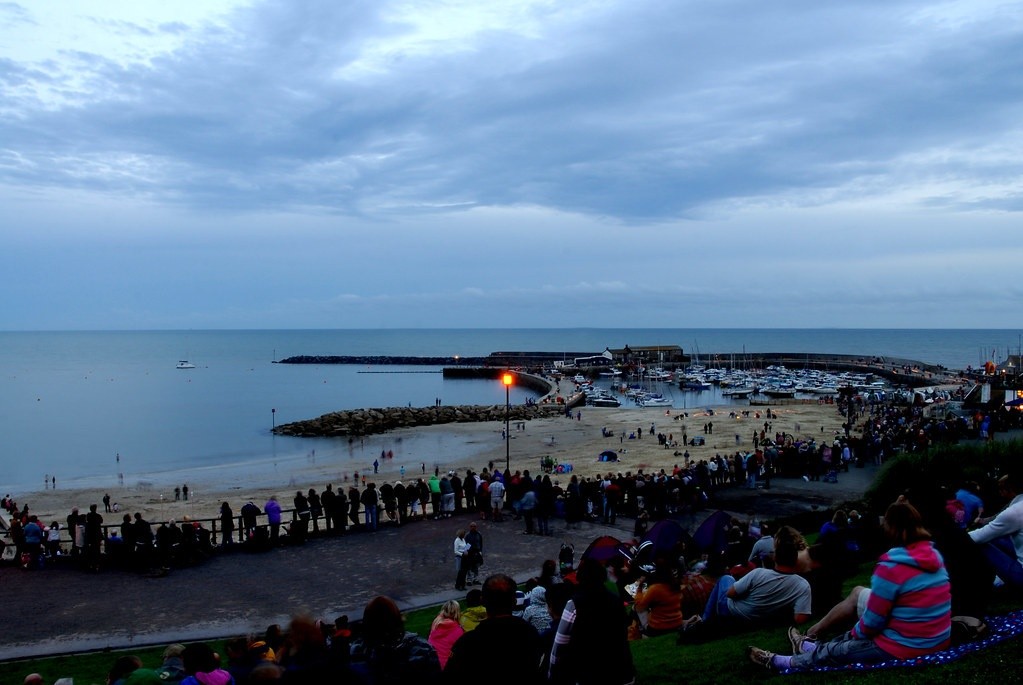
[503,375,512,472]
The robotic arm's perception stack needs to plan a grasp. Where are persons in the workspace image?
[0,494,234,578]
[435,398,442,406]
[502,384,614,442]
[348,437,353,445]
[23,542,636,685]
[183,484,189,500]
[624,471,1023,676]
[45,474,55,484]
[174,485,181,500]
[117,453,120,461]
[409,401,411,407]
[624,399,1023,537]
[241,450,624,541]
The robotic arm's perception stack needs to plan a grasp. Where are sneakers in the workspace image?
[745,646,776,669]
[788,625,818,656]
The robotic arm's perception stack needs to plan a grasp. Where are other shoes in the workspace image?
[462,587,467,590]
[466,582,472,586]
[476,581,482,585]
[455,585,463,591]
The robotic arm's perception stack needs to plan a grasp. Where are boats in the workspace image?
[611,376,674,407]
[175,360,195,368]
[722,389,755,400]
[572,374,623,407]
[678,378,712,391]
[756,354,886,397]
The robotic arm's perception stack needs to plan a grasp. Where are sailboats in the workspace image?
[646,339,757,389]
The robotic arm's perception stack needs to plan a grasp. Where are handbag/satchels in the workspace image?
[950,616,995,642]
[628,619,643,642]
